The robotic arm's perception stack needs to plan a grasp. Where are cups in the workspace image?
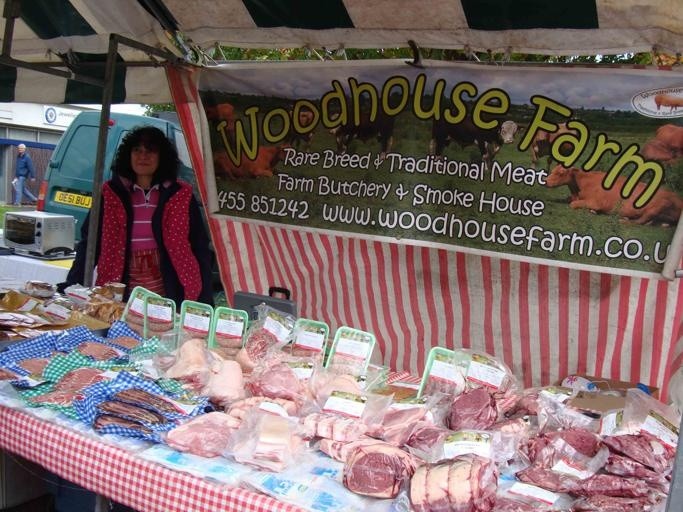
[105,280,126,301]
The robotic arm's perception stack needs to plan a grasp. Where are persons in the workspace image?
[11,143,38,206]
[58,124,219,312]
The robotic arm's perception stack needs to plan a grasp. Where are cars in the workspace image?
[36,111,217,271]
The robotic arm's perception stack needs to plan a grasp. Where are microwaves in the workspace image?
[3,209,76,259]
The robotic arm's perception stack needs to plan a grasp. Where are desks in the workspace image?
[1,282,423,512]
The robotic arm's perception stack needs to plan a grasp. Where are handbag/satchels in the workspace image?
[12,178,18,190]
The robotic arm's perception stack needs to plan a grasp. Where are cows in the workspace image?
[530,117,584,171]
[286,109,319,154]
[429,107,524,171]
[328,112,403,159]
[544,162,682,228]
[214,142,296,182]
